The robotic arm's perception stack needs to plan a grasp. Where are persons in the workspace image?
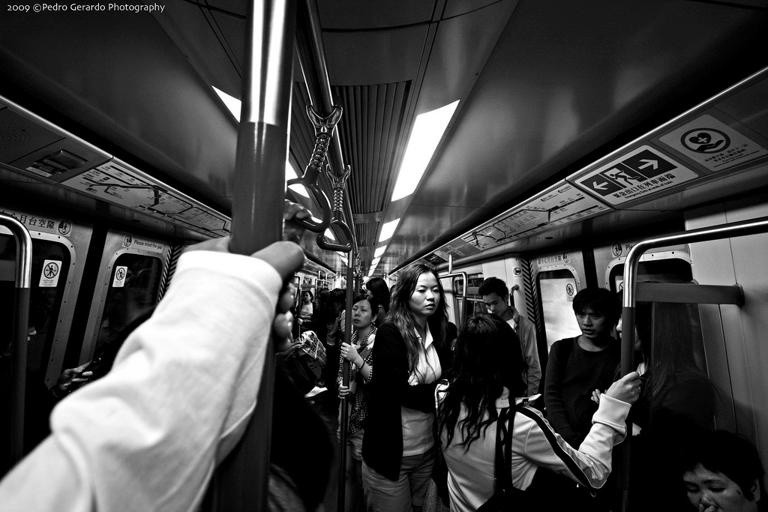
[0,231,306,511]
[268,271,765,511]
